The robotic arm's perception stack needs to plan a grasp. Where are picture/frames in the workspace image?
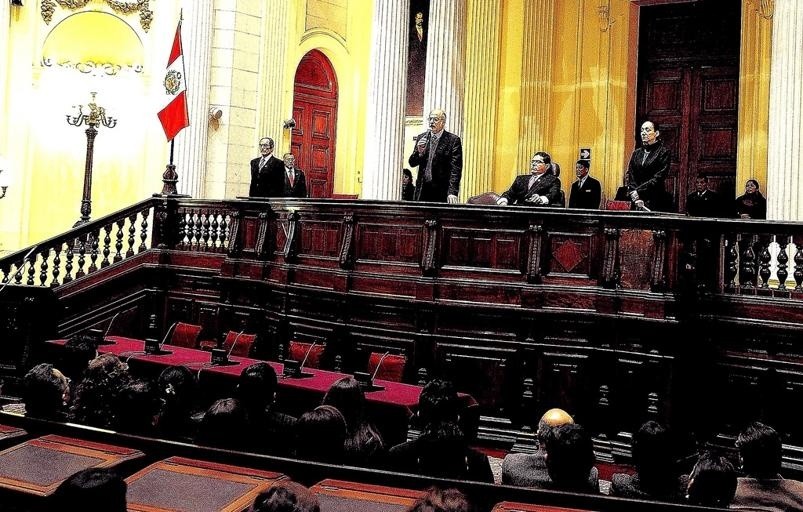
[406,0,431,127]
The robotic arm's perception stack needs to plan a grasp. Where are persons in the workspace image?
[249,138,285,196]
[496,149,561,206]
[568,160,602,209]
[407,10,427,72]
[623,119,673,211]
[401,169,414,201]
[614,171,633,201]
[733,178,767,220]
[280,153,306,196]
[684,174,718,215]
[19,354,801,512]
[408,107,464,202]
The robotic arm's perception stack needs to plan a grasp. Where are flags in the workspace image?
[155,21,192,145]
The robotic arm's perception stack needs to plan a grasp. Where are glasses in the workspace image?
[62,377,71,392]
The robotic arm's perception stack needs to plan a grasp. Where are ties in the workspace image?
[425,136,438,182]
[259,159,265,172]
[528,176,537,190]
[289,170,294,188]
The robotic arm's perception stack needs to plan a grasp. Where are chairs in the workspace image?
[170,321,201,347]
[222,329,258,358]
[606,200,631,211]
[288,340,325,368]
[367,352,407,381]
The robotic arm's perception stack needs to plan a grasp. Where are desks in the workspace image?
[490,498,597,512]
[46,335,478,441]
[114,455,291,512]
[302,478,429,511]
[1,431,146,496]
[1,422,28,442]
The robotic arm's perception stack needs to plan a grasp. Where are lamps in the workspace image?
[64,84,117,255]
[283,117,296,130]
[0,168,10,201]
[207,105,223,121]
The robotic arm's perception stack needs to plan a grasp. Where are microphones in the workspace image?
[421,127,432,148]
[353,349,391,391]
[144,321,180,354]
[282,339,317,379]
[86,310,123,345]
[211,327,246,365]
